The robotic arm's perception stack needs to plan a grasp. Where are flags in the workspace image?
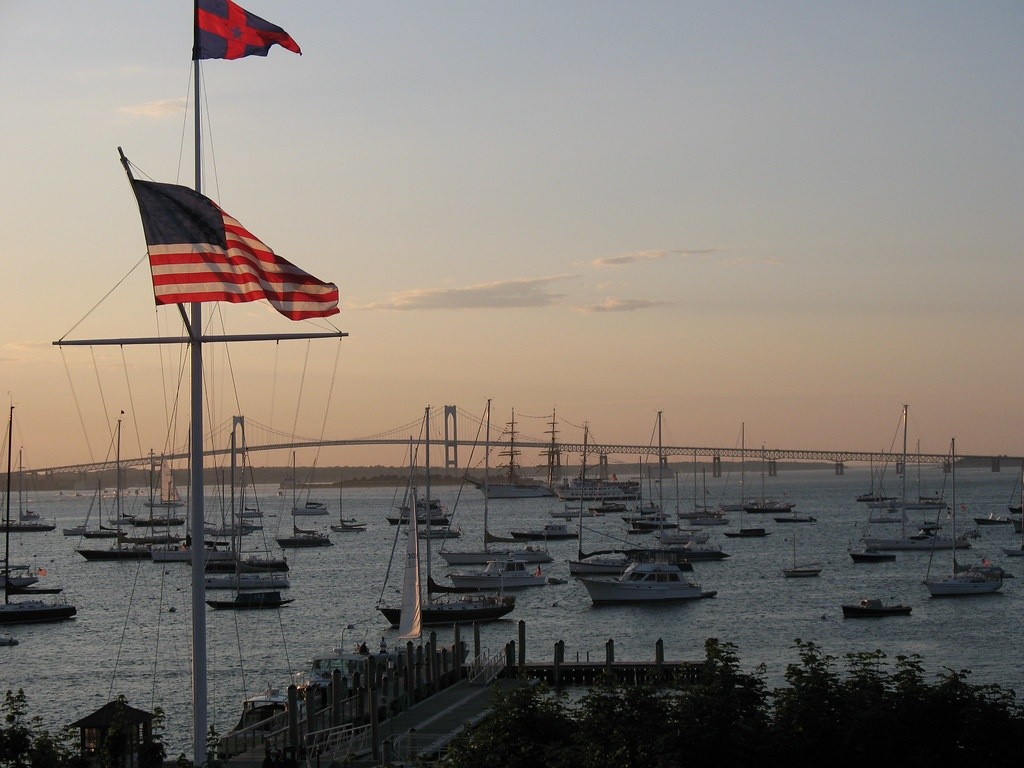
[38,567,47,576]
[132,179,340,321]
[195,0,303,60]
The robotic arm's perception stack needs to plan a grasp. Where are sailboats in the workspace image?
[922,437,1005,596]
[972,459,1024,555]
[377,399,797,629]
[0,406,77,644]
[63,415,368,610]
[848,403,971,564]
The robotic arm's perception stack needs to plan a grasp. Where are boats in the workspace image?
[782,533,821,576]
[772,509,816,523]
[842,596,911,617]
[578,560,715,601]
[215,482,467,759]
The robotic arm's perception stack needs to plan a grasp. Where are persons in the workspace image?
[537,565,541,576]
[359,641,367,654]
[380,638,387,654]
